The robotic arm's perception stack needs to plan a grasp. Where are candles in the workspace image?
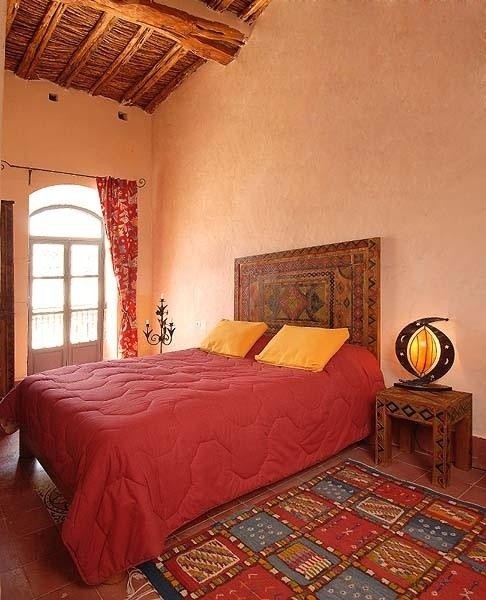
[169,318,173,322]
[160,292,164,299]
[146,320,150,324]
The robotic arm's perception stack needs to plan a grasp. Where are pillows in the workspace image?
[254,325,350,372]
[199,319,267,358]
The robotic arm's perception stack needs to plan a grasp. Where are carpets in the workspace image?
[126,460,486,600]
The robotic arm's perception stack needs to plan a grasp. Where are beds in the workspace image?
[0,238,381,586]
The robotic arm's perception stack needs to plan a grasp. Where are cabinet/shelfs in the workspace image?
[0,200,15,398]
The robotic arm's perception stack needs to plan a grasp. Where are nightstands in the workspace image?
[375,386,472,489]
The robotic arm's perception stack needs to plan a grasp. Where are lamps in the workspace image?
[394,318,455,392]
